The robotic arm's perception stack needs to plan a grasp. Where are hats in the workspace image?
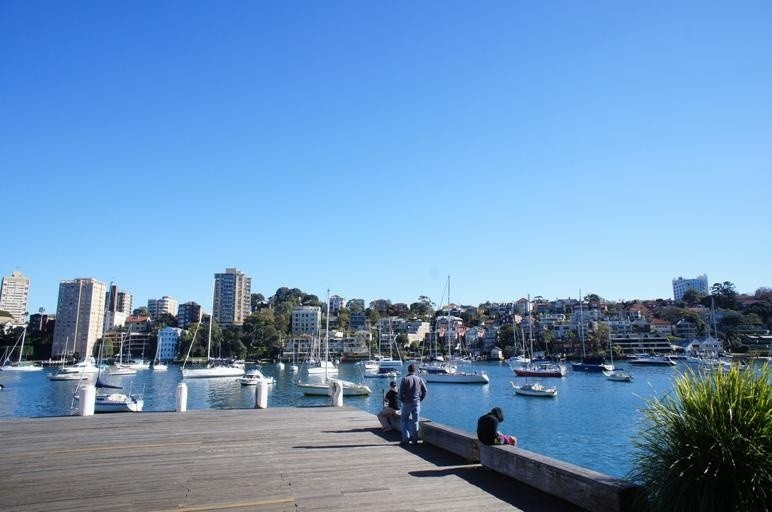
[408,365,416,372]
[390,381,396,386]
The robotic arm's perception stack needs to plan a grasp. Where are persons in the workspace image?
[397,364,429,447]
[476,408,518,447]
[376,380,401,433]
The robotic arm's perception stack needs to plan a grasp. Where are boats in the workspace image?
[70,391,145,412]
[289,365,298,371]
[237,367,275,385]
[625,355,678,365]
[276,361,285,369]
[509,381,558,397]
[686,357,748,375]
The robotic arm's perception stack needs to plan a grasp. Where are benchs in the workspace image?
[388,411,648,512]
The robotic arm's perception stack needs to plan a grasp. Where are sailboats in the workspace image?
[179,279,246,379]
[502,294,567,377]
[568,290,616,373]
[48,280,167,382]
[296,273,489,396]
[1,324,44,371]
[601,322,631,381]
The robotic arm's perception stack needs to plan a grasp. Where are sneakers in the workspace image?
[399,441,417,447]
[379,426,392,431]
